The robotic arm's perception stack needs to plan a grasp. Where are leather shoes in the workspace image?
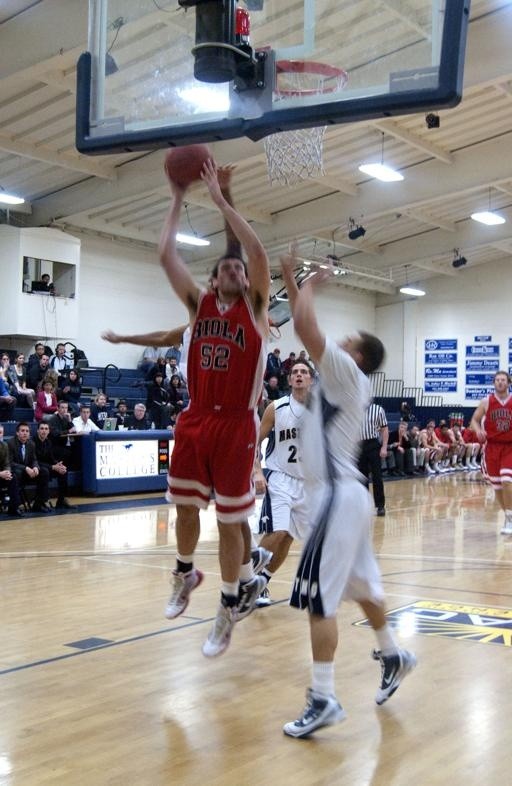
[56,501,77,509]
[7,508,25,516]
[32,504,53,512]
[390,470,421,476]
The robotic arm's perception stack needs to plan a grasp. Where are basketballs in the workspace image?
[165,142,211,186]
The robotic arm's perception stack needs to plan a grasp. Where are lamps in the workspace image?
[346,215,367,242]
[451,245,469,269]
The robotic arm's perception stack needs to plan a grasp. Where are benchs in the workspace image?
[0,346,441,499]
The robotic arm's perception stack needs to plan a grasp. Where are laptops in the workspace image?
[103,418,117,430]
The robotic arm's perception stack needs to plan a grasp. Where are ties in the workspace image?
[22,445,25,461]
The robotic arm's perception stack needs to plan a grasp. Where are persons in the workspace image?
[143,344,190,430]
[280,238,417,737]
[358,401,389,517]
[41,273,50,290]
[73,392,155,434]
[158,156,270,656]
[386,418,482,475]
[469,370,512,538]
[258,349,311,418]
[102,163,274,621]
[253,361,315,606]
[2,344,82,518]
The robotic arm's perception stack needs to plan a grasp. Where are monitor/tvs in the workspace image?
[32,281,46,290]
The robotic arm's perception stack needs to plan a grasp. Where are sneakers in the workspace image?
[284,689,345,737]
[501,520,512,535]
[425,463,481,474]
[377,507,385,515]
[166,568,202,619]
[202,546,273,656]
[373,649,415,704]
